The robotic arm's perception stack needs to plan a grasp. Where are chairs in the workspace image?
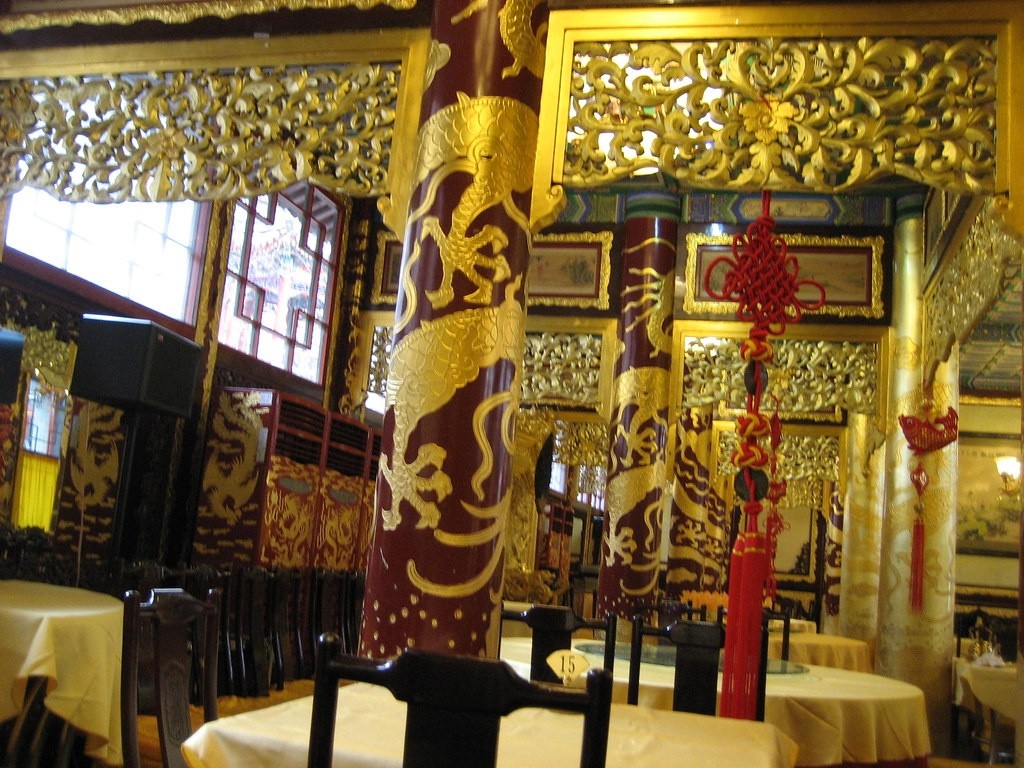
[0,568,1023,768]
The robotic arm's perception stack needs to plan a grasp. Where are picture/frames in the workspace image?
[955,430,1020,558]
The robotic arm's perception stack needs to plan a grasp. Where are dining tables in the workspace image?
[178,674,801,767]
[1,578,124,767]
[498,636,933,767]
[720,631,872,674]
[950,654,1023,765]
[766,618,817,634]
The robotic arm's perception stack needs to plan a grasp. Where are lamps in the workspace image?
[993,454,1023,501]
